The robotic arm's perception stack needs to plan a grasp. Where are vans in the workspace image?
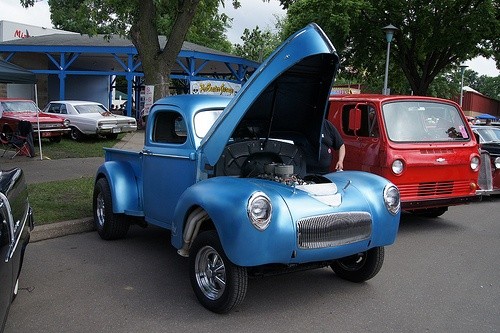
[320,93,482,224]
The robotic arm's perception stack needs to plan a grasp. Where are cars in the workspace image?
[0,164,36,333]
[40,100,137,141]
[0,97,72,147]
[471,124,500,159]
[91,23,403,317]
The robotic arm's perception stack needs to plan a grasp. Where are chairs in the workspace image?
[0,120,34,159]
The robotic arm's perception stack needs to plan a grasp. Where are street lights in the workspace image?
[382,23,400,96]
[458,65,469,107]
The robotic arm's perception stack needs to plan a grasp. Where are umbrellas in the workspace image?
[475,113,496,119]
[465,116,474,119]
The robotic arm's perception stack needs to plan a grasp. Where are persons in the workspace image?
[307,119,346,174]
